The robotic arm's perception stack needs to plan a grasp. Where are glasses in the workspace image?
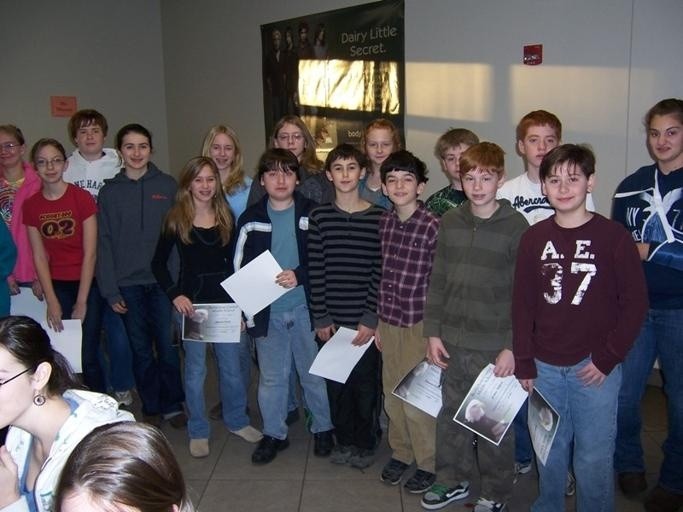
[278,133,301,142]
[0,368,31,386]
[0,143,22,149]
[36,158,64,165]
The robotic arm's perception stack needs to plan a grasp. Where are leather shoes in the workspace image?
[231,426,264,443]
[190,438,210,457]
[252,435,289,464]
[313,432,334,456]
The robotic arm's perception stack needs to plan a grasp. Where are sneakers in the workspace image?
[421,481,470,510]
[143,413,161,428]
[380,459,409,485]
[114,391,134,409]
[616,471,647,495]
[514,461,532,474]
[349,449,375,468]
[644,487,682,512]
[170,414,188,429]
[330,445,356,465]
[566,474,575,495]
[472,497,505,512]
[404,469,437,493]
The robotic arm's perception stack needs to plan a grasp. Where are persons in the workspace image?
[202,125,259,228]
[0,208,18,321]
[97,122,191,431]
[424,127,481,223]
[62,108,136,409]
[1,124,46,303]
[373,149,441,494]
[49,421,193,512]
[151,156,261,457]
[1,315,137,512]
[229,148,334,467]
[421,142,532,511]
[512,144,648,512]
[22,137,107,395]
[307,144,386,467]
[357,120,401,209]
[612,98,682,512]
[495,110,595,226]
[247,115,328,207]
